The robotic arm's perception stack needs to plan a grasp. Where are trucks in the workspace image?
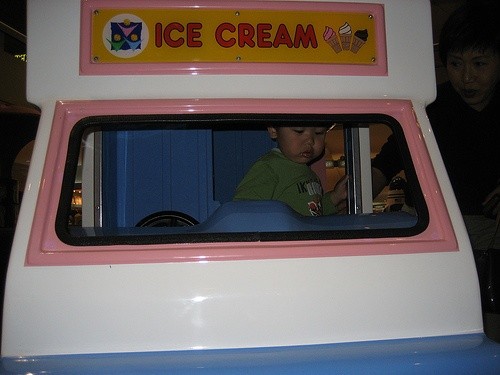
[2,0,499,375]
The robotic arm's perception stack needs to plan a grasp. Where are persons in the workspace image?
[231,124,350,219]
[373,0,500,343]
